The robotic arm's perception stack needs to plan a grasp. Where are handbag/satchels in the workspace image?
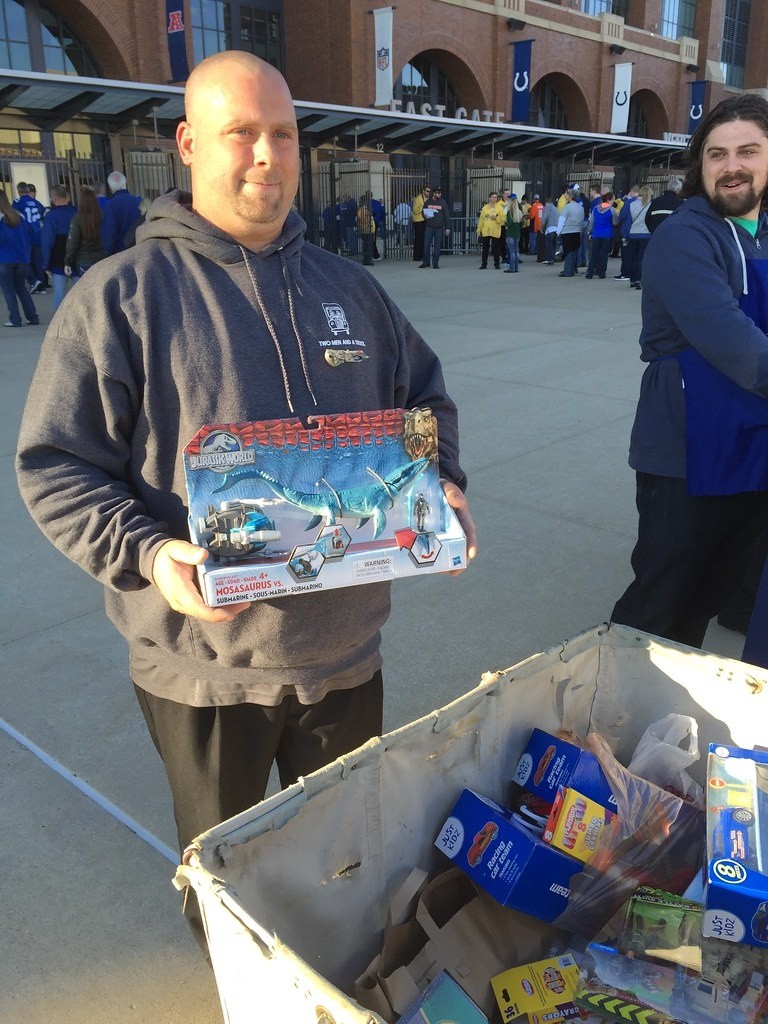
[553,733,706,933]
[355,867,558,1024]
[479,235,482,242]
[629,713,704,809]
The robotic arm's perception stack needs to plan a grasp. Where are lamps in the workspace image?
[686,63,700,73]
[506,17,526,31]
[609,44,626,55]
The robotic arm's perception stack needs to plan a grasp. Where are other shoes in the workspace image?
[635,283,642,289]
[630,283,636,287]
[505,270,511,272]
[372,257,380,261]
[480,265,486,269]
[495,265,500,269]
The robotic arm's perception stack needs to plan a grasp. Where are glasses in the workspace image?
[425,190,430,192]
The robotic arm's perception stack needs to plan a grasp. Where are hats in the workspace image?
[569,184,580,190]
[434,186,444,191]
[508,193,517,199]
[534,194,539,198]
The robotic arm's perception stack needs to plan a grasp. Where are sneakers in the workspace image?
[419,264,430,267]
[35,288,46,294]
[433,264,439,268]
[542,260,553,263]
[3,322,22,327]
[29,279,41,294]
[614,274,630,280]
[25,320,39,325]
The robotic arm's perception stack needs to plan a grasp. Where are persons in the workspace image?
[394,185,450,269]
[16,50,477,970]
[322,190,386,266]
[0,171,178,326]
[475,179,687,290]
[610,95,768,650]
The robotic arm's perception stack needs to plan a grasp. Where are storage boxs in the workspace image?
[182,407,469,608]
[433,788,584,923]
[617,886,704,974]
[543,784,650,886]
[700,743,768,949]
[571,900,768,1024]
[172,620,768,1024]
[489,954,590,1024]
[510,727,706,829]
